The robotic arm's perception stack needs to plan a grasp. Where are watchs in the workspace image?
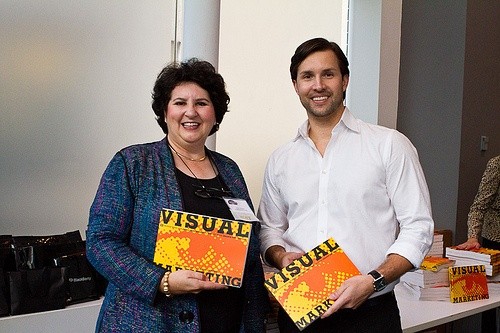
[369,269,387,292]
[163,271,173,298]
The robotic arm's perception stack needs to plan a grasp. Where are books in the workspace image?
[423,229,452,259]
[153,208,252,288]
[445,246,500,277]
[448,264,489,303]
[263,238,363,331]
[399,257,456,301]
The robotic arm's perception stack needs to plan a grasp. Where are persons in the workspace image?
[257,37,434,333]
[456,156,500,250]
[86,59,261,333]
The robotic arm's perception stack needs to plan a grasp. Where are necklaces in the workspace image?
[167,141,224,199]
[178,151,207,161]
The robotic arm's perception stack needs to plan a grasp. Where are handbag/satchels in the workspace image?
[0,229,107,319]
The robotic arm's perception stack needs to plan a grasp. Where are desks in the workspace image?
[0,280,500,333]
